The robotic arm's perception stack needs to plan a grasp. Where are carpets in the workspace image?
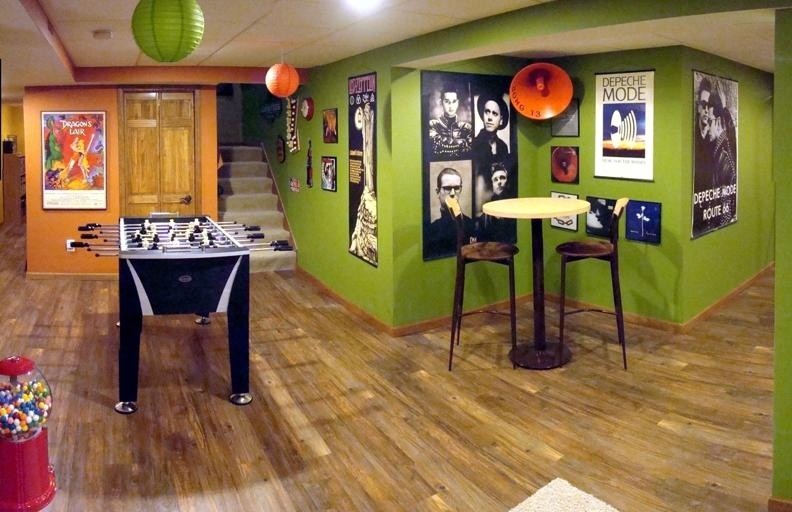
[507,477,621,512]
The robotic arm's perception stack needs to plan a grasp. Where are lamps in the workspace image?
[265,43,300,99]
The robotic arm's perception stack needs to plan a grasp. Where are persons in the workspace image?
[359,102,377,199]
[474,96,514,154]
[306,140,313,187]
[480,162,515,237]
[320,157,336,191]
[695,78,737,232]
[707,94,735,222]
[428,87,473,155]
[430,167,471,244]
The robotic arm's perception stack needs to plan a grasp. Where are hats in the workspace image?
[477,91,509,130]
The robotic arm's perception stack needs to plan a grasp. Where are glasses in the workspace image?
[441,186,461,194]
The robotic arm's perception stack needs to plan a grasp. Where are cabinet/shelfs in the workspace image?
[3,154,26,226]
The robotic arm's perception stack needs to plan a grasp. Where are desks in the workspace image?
[482,197,591,369]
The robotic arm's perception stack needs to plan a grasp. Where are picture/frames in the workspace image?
[322,108,338,143]
[586,196,618,237]
[321,155,337,192]
[626,200,661,244]
[550,98,580,138]
[551,189,579,232]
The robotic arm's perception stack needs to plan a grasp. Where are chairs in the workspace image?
[445,195,519,371]
[556,197,630,372]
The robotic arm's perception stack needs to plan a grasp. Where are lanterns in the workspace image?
[264,64,302,99]
